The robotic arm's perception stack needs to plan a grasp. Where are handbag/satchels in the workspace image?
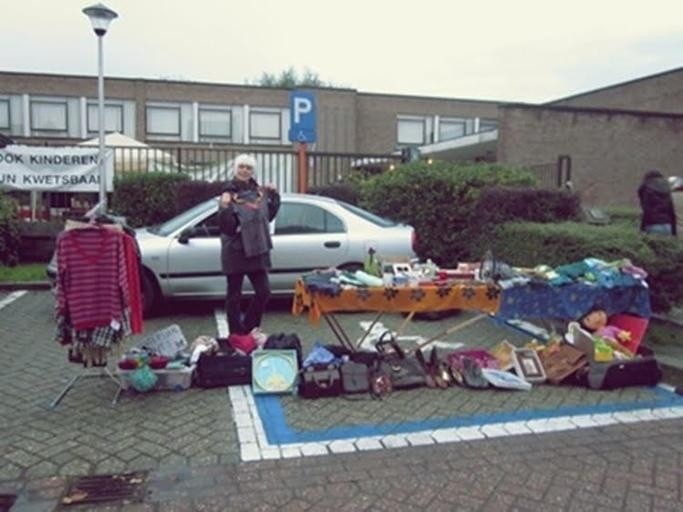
[262,334,302,367]
[299,330,425,399]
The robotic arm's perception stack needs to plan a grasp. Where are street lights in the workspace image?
[83,0,126,218]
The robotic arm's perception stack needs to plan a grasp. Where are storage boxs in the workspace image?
[120,364,194,390]
[566,360,660,390]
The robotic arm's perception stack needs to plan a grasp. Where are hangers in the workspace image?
[80,197,113,234]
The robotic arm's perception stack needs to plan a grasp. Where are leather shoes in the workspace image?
[417,347,488,388]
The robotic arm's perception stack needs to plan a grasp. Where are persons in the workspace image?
[635,170,673,236]
[216,154,280,337]
[666,176,682,237]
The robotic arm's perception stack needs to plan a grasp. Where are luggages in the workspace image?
[565,346,662,388]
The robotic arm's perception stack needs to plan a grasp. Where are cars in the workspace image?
[43,192,423,314]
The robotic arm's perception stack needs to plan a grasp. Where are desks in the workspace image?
[289,265,649,357]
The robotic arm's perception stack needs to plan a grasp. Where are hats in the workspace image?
[666,176,682,192]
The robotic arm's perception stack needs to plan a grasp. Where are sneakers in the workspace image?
[249,327,267,346]
